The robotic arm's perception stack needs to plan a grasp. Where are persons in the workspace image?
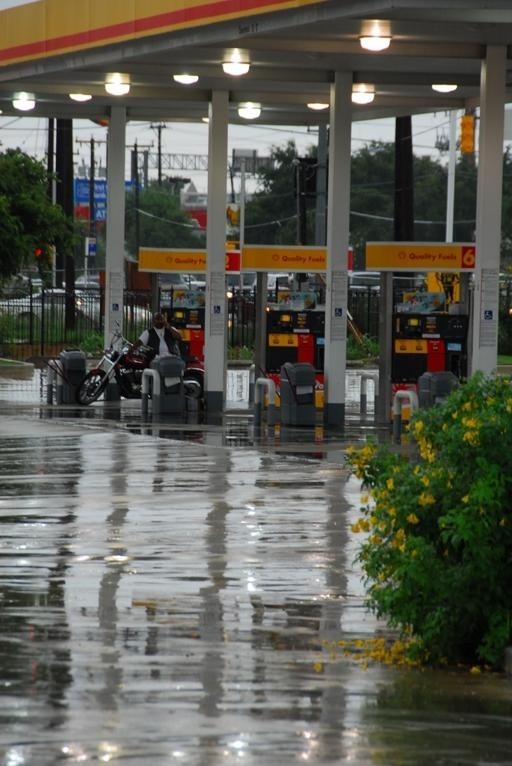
[126,309,182,373]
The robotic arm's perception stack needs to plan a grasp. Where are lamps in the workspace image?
[173,74,199,85]
[12,100,36,112]
[352,93,376,106]
[104,83,130,97]
[432,84,458,93]
[221,61,250,76]
[70,94,93,102]
[360,36,392,51]
[238,108,261,121]
[306,103,328,111]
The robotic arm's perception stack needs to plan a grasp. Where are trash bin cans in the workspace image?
[280,363,316,426]
[150,354,186,415]
[60,348,86,404]
[418,372,460,408]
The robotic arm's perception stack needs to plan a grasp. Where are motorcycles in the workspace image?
[75,320,206,407]
